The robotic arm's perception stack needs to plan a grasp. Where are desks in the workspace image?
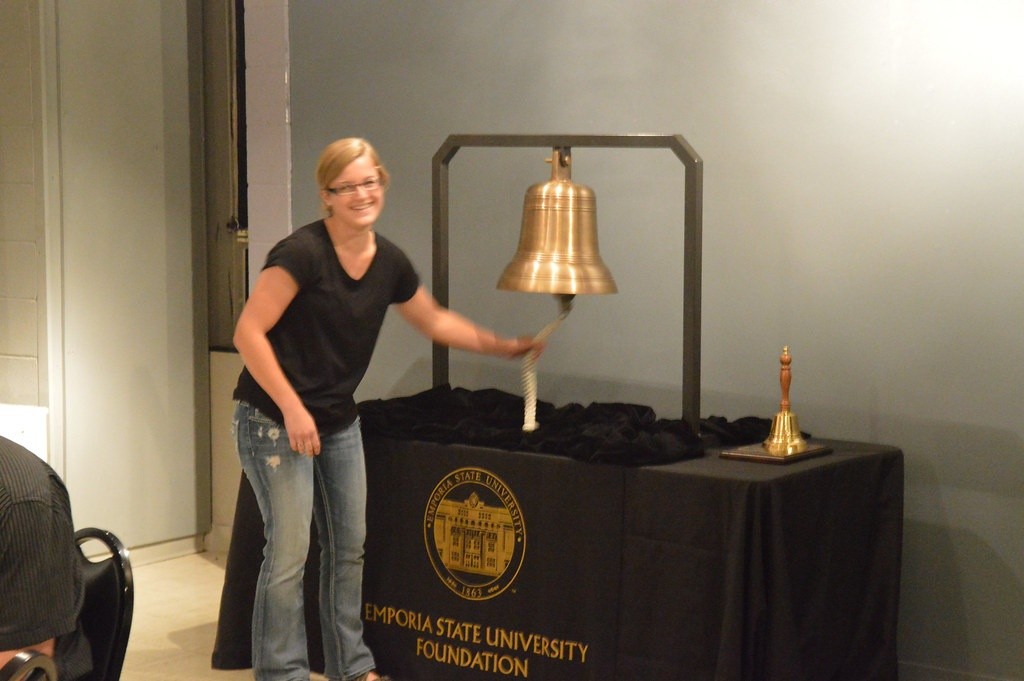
[211,388,904,680]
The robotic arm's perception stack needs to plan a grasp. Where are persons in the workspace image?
[230,137,547,681]
[0,435,95,681]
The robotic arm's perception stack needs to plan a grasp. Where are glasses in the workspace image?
[328,177,382,195]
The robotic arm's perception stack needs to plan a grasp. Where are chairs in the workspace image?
[0,523,135,681]
